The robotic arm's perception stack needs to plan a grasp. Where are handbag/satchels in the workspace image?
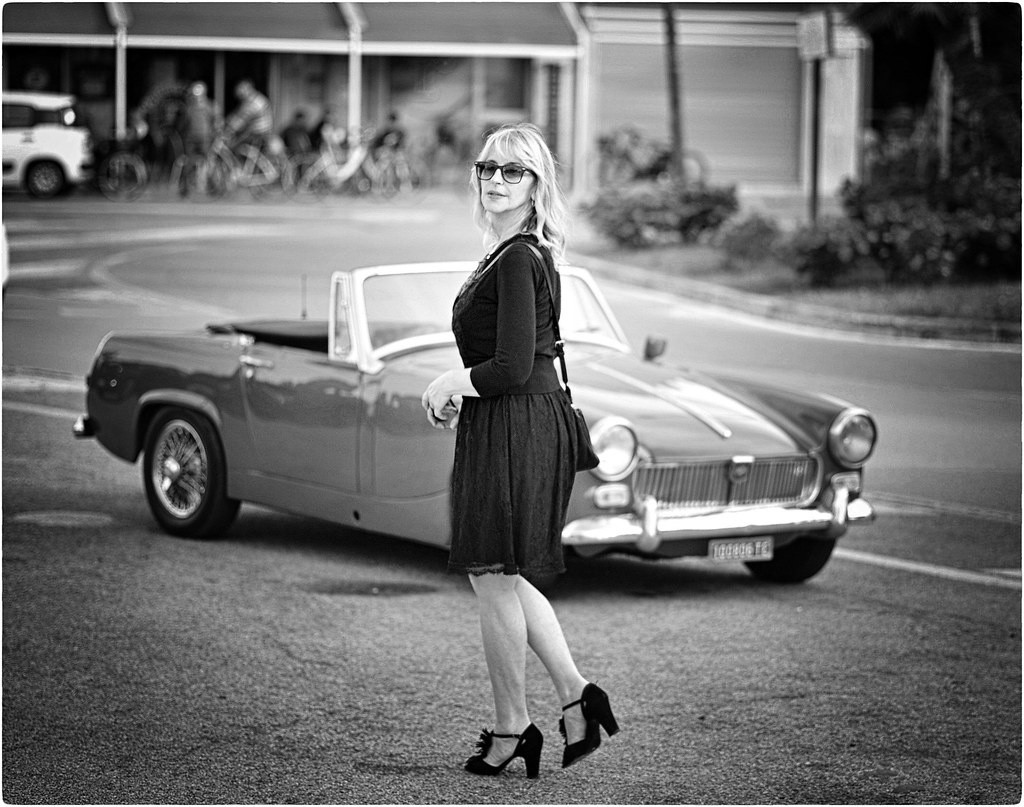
[573,408,600,471]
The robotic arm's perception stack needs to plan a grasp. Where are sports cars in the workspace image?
[69,262,880,595]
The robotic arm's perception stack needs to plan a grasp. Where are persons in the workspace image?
[420,121,621,780]
[172,82,410,200]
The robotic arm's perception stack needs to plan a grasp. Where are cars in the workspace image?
[2,91,93,199]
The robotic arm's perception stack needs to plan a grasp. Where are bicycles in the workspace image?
[97,127,433,208]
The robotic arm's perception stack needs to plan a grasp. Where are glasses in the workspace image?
[475,160,534,184]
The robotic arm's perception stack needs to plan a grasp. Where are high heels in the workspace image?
[465,722,544,779]
[559,683,620,768]
